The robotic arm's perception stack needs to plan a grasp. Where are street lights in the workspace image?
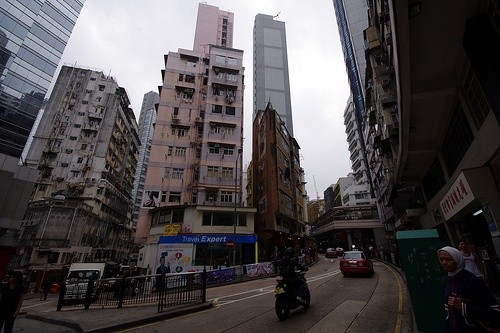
[233,148,243,266]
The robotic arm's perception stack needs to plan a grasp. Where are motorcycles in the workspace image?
[275,267,310,321]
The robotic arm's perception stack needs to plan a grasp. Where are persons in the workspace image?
[459,240,484,280]
[0,271,26,333]
[279,247,308,298]
[437,246,490,333]
[156,256,168,274]
[367,243,404,270]
[39,287,49,301]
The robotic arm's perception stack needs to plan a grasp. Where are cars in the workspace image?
[335,247,344,256]
[325,248,337,257]
[339,250,374,275]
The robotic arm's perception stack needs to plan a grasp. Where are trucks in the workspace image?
[62,263,107,302]
